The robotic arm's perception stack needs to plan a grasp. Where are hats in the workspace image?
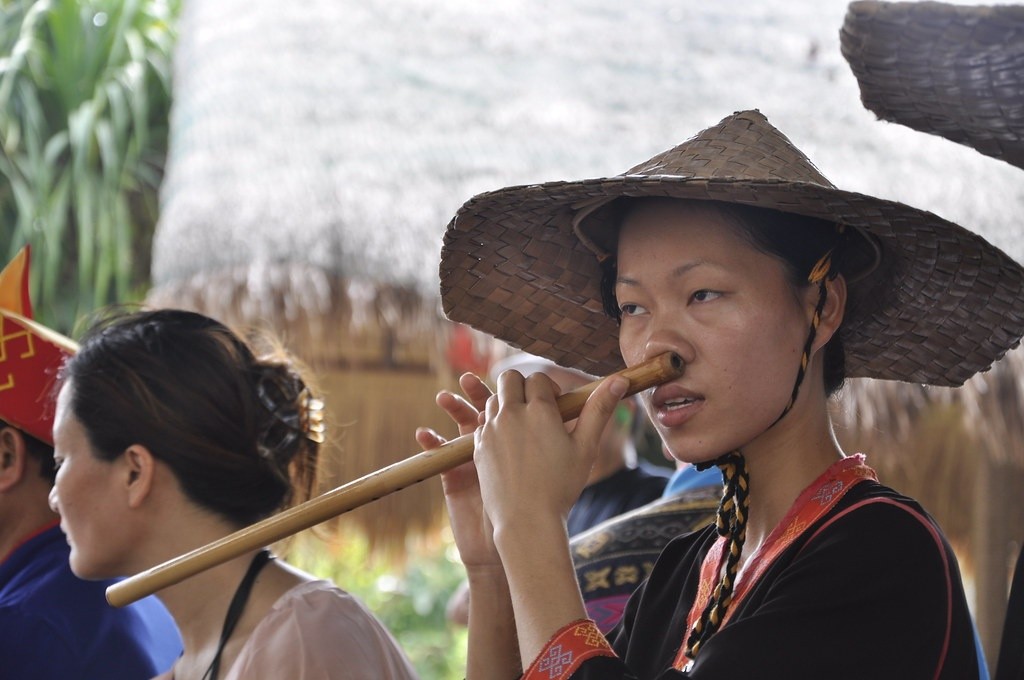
[0,243,87,447]
[488,345,605,390]
[439,109,1024,389]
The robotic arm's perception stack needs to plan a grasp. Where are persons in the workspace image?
[415,110,991,680]
[445,351,727,640]
[48,308,417,680]
[0,309,185,680]
